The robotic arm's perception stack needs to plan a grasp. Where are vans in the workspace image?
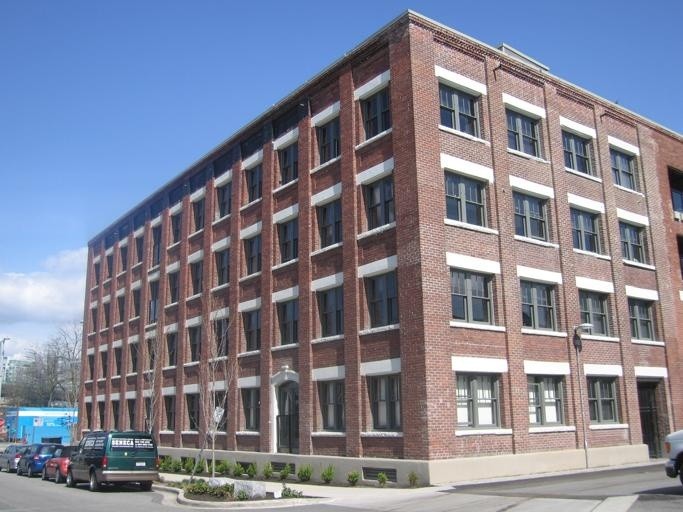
[66,432,159,491]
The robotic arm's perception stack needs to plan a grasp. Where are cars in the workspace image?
[0,443,78,483]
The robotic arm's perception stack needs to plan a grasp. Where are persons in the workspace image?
[23,436,26,444]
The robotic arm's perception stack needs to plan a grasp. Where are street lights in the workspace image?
[573,323,594,469]
